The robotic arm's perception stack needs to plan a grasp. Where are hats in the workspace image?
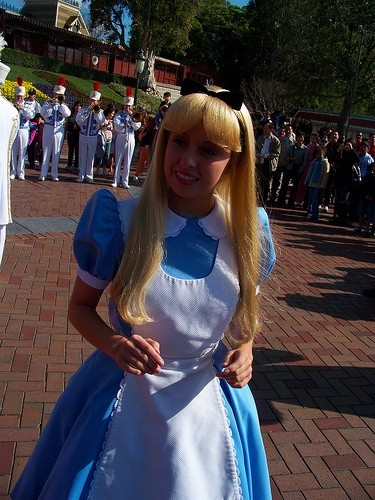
[89,81,101,101]
[28,88,36,95]
[361,140,369,147]
[344,137,354,148]
[123,87,134,106]
[52,75,67,94]
[0,32,11,84]
[14,76,26,96]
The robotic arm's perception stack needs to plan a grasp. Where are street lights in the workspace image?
[133,49,146,109]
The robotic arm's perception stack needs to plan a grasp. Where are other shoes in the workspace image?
[53,177,59,181]
[95,170,103,176]
[70,166,77,170]
[132,176,138,181]
[260,195,328,219]
[28,164,37,169]
[75,178,84,182]
[103,170,109,175]
[86,178,95,184]
[122,184,130,188]
[38,176,44,181]
[10,174,15,180]
[18,175,25,181]
[64,166,71,169]
[111,183,120,187]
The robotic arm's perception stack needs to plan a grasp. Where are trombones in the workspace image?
[124,108,143,143]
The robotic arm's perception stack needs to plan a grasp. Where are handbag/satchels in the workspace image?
[350,163,362,184]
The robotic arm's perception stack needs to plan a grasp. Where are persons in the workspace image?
[10,76,35,183]
[0,32,19,259]
[255,118,375,237]
[13,83,277,500]
[110,88,142,189]
[25,88,41,166]
[75,81,105,183]
[38,75,71,181]
[95,92,172,177]
[65,100,81,171]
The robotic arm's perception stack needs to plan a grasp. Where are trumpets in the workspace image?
[91,100,103,113]
[55,94,68,106]
